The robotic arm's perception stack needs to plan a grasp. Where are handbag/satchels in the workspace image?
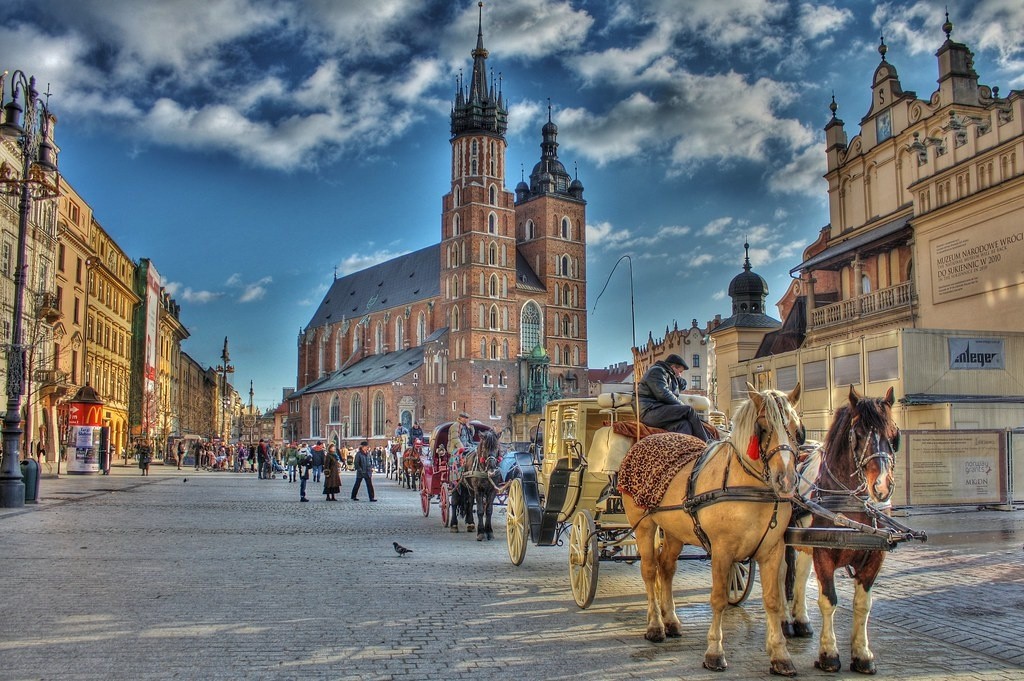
[323,469,331,477]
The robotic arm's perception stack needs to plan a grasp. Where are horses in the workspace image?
[450,428,505,541]
[618,382,806,677]
[402,436,424,492]
[392,443,413,482]
[776,382,901,674]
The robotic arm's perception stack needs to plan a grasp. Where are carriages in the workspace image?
[419,420,504,542]
[385,435,430,490]
[504,380,926,677]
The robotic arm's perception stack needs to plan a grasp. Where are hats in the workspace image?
[398,423,403,426]
[459,412,470,418]
[665,354,689,371]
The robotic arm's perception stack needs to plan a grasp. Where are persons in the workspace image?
[631,353,710,444]
[60,421,424,502]
[447,412,475,460]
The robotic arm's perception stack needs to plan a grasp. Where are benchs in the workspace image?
[597,392,729,431]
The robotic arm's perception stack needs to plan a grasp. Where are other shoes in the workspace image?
[351,497,359,501]
[372,498,377,501]
[325,497,338,501]
[300,497,308,502]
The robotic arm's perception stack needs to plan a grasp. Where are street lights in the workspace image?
[215,364,234,442]
[0,69,58,508]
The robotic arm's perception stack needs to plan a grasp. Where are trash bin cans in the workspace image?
[20,458,40,504]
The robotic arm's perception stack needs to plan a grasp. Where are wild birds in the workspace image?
[392,541,415,557]
[183,477,187,482]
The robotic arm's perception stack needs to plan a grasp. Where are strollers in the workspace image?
[213,457,227,471]
[270,457,287,479]
